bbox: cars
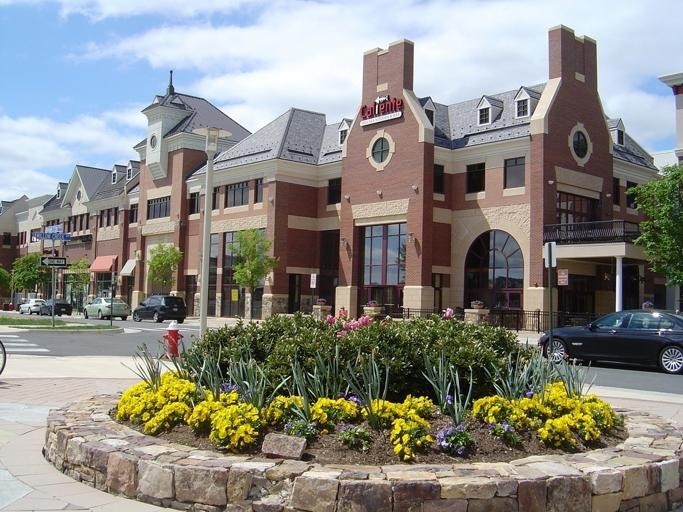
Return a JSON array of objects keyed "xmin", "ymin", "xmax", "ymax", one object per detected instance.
[
  {"xmin": 17, "ymin": 299, "xmax": 45, "ymax": 314},
  {"xmin": 40, "ymin": 298, "xmax": 71, "ymax": 316},
  {"xmin": 536, "ymin": 308, "xmax": 683, "ymax": 373},
  {"xmin": 82, "ymin": 295, "xmax": 127, "ymax": 322}
]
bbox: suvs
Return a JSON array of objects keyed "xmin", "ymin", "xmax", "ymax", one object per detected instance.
[{"xmin": 132, "ymin": 295, "xmax": 186, "ymax": 324}]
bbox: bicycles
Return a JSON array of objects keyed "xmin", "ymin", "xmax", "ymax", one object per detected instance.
[{"xmin": 0, "ymin": 339, "xmax": 5, "ymax": 376}]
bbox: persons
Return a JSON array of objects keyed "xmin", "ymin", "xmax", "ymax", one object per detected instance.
[{"xmin": 641, "ymin": 296, "xmax": 654, "ymax": 310}]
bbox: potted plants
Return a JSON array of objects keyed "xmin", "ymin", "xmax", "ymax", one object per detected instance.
[
  {"xmin": 368, "ymin": 301, "xmax": 378, "ymax": 306},
  {"xmin": 471, "ymin": 300, "xmax": 484, "ymax": 309},
  {"xmin": 316, "ymin": 299, "xmax": 327, "ymax": 306}
]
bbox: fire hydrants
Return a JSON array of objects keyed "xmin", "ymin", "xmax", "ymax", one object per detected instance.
[
  {"xmin": 163, "ymin": 319, "xmax": 183, "ymax": 357},
  {"xmin": 7, "ymin": 302, "xmax": 13, "ymax": 313}
]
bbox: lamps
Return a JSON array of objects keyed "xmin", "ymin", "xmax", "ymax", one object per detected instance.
[
  {"xmin": 408, "ymin": 231, "xmax": 416, "ymax": 243},
  {"xmin": 340, "ymin": 237, "xmax": 348, "ymax": 247}
]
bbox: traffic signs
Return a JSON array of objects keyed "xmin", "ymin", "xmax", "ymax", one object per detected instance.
[{"xmin": 40, "ymin": 257, "xmax": 65, "ymax": 267}]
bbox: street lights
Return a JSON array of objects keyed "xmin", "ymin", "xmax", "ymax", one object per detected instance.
[{"xmin": 192, "ymin": 124, "xmax": 234, "ymax": 341}]
